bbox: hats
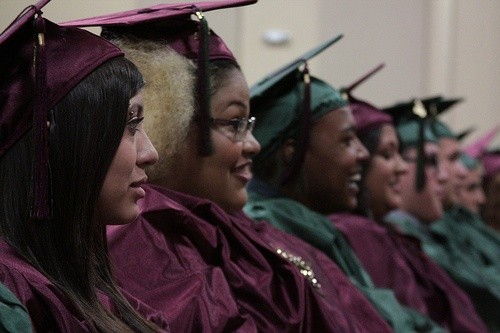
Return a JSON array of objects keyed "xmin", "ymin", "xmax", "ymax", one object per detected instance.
[{"xmin": 1, "ymin": 0, "xmax": 500, "ymax": 185}]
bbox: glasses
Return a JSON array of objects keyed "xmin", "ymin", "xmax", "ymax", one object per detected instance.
[{"xmin": 208, "ymin": 116, "xmax": 257, "ymax": 134}]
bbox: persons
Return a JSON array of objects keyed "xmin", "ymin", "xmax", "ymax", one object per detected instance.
[
  {"xmin": 243, "ymin": 35, "xmax": 500, "ymax": 333},
  {"xmin": 58, "ymin": 0, "xmax": 392, "ymax": 333},
  {"xmin": 0, "ymin": 0, "xmax": 172, "ymax": 333}
]
